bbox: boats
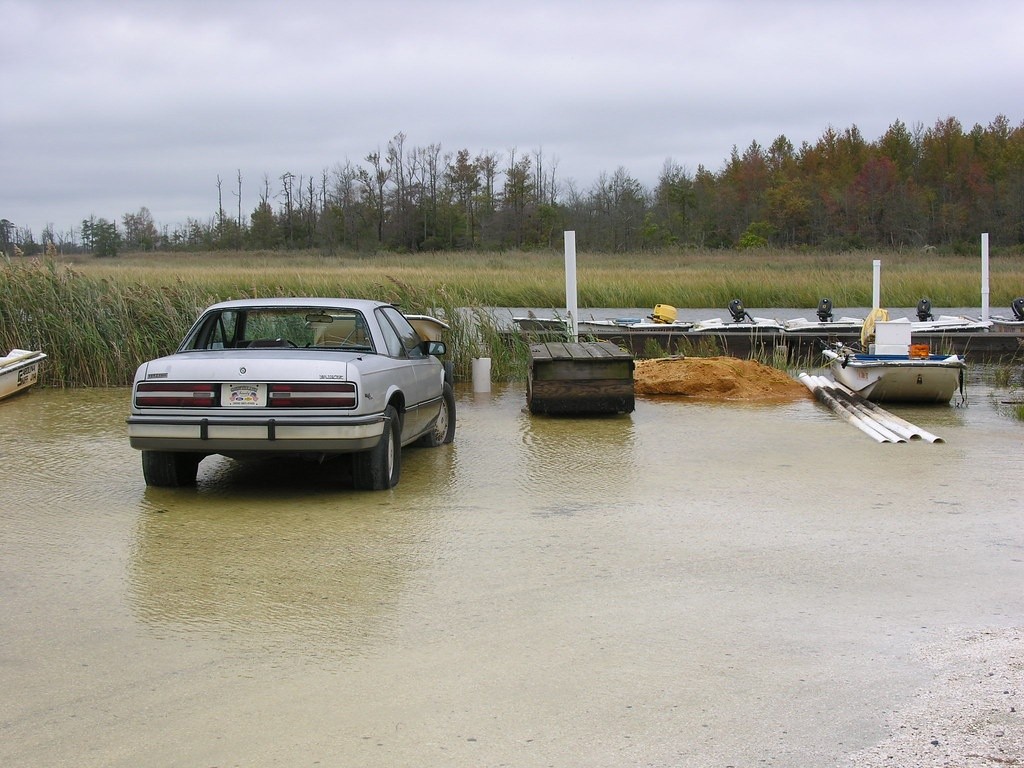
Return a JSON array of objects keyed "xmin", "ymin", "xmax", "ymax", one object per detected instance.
[
  {"xmin": 511, "ymin": 300, "xmax": 696, "ymax": 332},
  {"xmin": 691, "ymin": 297, "xmax": 785, "ymax": 333},
  {"xmin": 977, "ymin": 297, "xmax": 1024, "ymax": 333},
  {"xmin": 306, "ymin": 313, "xmax": 453, "ymax": 347},
  {"xmin": 781, "ymin": 297, "xmax": 866, "ymax": 334},
  {"xmin": 0, "ymin": 347, "xmax": 48, "ymax": 400},
  {"xmin": 910, "ymin": 298, "xmax": 994, "ymax": 333},
  {"xmin": 820, "ymin": 317, "xmax": 968, "ymax": 404}
]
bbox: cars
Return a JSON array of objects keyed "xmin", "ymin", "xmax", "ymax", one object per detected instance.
[{"xmin": 122, "ymin": 295, "xmax": 458, "ymax": 492}]
